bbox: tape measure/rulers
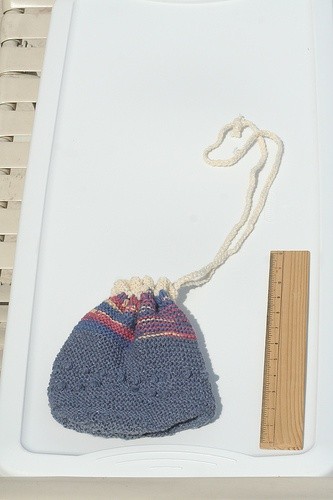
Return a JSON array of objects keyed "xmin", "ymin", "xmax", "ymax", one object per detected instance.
[{"xmin": 259, "ymin": 249, "xmax": 312, "ymax": 451}]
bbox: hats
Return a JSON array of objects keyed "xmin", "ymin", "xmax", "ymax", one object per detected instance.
[{"xmin": 45, "ymin": 112, "xmax": 284, "ymax": 438}]
